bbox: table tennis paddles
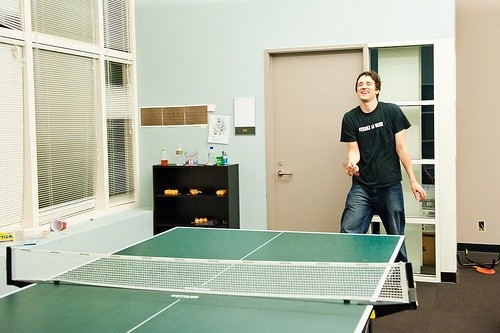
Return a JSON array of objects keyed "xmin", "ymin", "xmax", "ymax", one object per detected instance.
[
  {"xmin": 343, "ymin": 162, "xmax": 360, "ymax": 176},
  {"xmin": 473, "ymin": 266, "xmax": 496, "ymax": 274}
]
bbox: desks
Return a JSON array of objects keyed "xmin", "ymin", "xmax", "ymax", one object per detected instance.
[{"xmin": 0, "ymin": 226, "xmax": 419, "ymax": 333}]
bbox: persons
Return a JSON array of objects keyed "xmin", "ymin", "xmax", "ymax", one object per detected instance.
[{"xmin": 338, "ymin": 71, "xmax": 427, "ymax": 307}]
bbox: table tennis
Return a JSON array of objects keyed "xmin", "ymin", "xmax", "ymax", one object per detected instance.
[
  {"xmin": 165, "ymin": 190, "xmax": 178, "ymax": 195},
  {"xmin": 195, "ymin": 218, "xmax": 208, "ymax": 223},
  {"xmin": 216, "ymin": 189, "xmax": 226, "ymax": 196},
  {"xmin": 190, "ymin": 189, "xmax": 198, "ymax": 195}
]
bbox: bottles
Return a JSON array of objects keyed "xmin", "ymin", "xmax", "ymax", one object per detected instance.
[
  {"xmin": 175, "ymin": 145, "xmax": 184, "ymax": 166},
  {"xmin": 160, "ymin": 147, "xmax": 168, "ymax": 166},
  {"xmin": 208, "ymin": 146, "xmax": 216, "ymax": 165}
]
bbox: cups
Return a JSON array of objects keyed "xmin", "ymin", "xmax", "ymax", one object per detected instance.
[{"xmin": 50, "ymin": 219, "xmax": 67, "ymax": 232}]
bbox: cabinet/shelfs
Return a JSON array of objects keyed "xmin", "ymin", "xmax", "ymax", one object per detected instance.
[{"xmin": 153, "ymin": 164, "xmax": 240, "ymax": 235}]
